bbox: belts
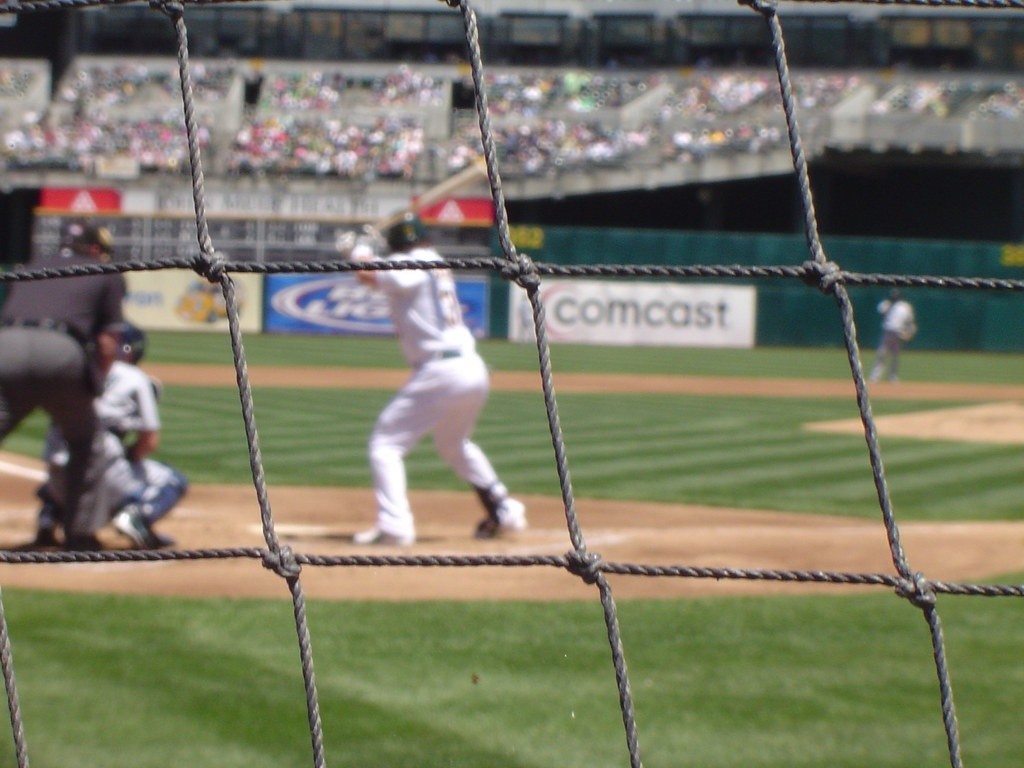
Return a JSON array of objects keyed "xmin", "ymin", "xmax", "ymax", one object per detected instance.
[
  {"xmin": 443, "ymin": 349, "xmax": 460, "ymax": 359},
  {"xmin": 0, "ymin": 318, "xmax": 87, "ymax": 349}
]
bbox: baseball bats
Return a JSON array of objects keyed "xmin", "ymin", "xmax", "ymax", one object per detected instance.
[{"xmin": 374, "ymin": 154, "xmax": 498, "ymax": 232}]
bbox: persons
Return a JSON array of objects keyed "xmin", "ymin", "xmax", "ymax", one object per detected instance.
[
  {"xmin": 870, "ymin": 288, "xmax": 918, "ymax": 384},
  {"xmin": 347, "ymin": 212, "xmax": 525, "ymax": 550},
  {"xmin": 0, "ymin": 216, "xmax": 128, "ymax": 549},
  {"xmin": 0, "ymin": 62, "xmax": 236, "ymax": 174},
  {"xmin": 33, "ymin": 319, "xmax": 190, "ymax": 550},
  {"xmin": 797, "ymin": 74, "xmax": 1023, "ymax": 123},
  {"xmin": 449, "ymin": 70, "xmax": 800, "ymax": 183},
  {"xmin": 232, "ymin": 63, "xmax": 447, "ymax": 184}
]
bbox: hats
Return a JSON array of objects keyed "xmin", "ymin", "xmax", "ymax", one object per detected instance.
[{"xmin": 60, "ymin": 220, "xmax": 113, "ymax": 264}]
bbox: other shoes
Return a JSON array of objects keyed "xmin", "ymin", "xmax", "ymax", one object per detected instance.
[
  {"xmin": 114, "ymin": 502, "xmax": 172, "ymax": 550},
  {"xmin": 35, "ymin": 523, "xmax": 66, "ymax": 545},
  {"xmin": 476, "ymin": 513, "xmax": 526, "ymax": 539},
  {"xmin": 66, "ymin": 522, "xmax": 136, "ymax": 551},
  {"xmin": 354, "ymin": 526, "xmax": 415, "ymax": 546}
]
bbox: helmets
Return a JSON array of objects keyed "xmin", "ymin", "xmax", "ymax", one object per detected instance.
[
  {"xmin": 119, "ymin": 326, "xmax": 144, "ymax": 366},
  {"xmin": 387, "ymin": 216, "xmax": 425, "ymax": 250}
]
